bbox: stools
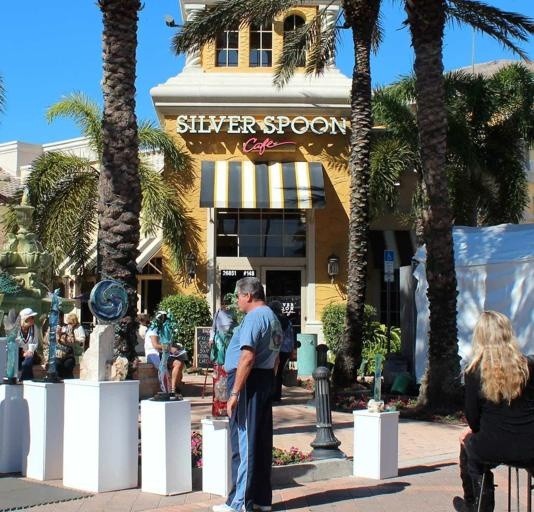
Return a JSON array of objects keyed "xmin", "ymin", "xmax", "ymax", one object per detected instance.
[{"xmin": 476, "ymin": 462, "xmax": 533, "ymax": 511}]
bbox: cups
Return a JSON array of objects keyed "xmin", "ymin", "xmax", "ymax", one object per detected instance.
[{"xmin": 28, "ymin": 344, "xmax": 35, "ymax": 352}]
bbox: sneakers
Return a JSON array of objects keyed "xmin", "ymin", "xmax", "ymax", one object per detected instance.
[{"xmin": 212, "ymin": 503, "xmax": 272, "ymax": 512}]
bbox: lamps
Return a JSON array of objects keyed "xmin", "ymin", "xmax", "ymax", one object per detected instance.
[
  {"xmin": 184, "ymin": 252, "xmax": 197, "ymax": 284},
  {"xmin": 327, "ymin": 252, "xmax": 339, "ymax": 284}
]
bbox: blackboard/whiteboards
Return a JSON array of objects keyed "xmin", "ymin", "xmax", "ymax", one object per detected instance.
[{"xmin": 195, "ymin": 326, "xmax": 215, "ymax": 371}]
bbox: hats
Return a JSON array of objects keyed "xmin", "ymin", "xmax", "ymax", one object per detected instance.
[{"xmin": 19, "ymin": 308, "xmax": 38, "ymax": 319}]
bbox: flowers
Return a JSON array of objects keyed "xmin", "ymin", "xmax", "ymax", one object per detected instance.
[
  {"xmin": 272, "ymin": 444, "xmax": 314, "ymax": 467},
  {"xmin": 191, "ymin": 429, "xmax": 202, "ymax": 469}
]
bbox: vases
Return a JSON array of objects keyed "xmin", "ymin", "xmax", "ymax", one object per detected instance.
[{"xmin": 270, "ymin": 464, "xmax": 315, "ymax": 486}]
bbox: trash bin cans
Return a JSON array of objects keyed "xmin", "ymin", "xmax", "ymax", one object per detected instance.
[{"xmin": 296, "ymin": 333, "xmax": 317, "ymax": 377}]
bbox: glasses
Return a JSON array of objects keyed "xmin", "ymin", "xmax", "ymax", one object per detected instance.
[
  {"xmin": 24, "ymin": 317, "xmax": 33, "ymax": 323},
  {"xmin": 236, "ymin": 294, "xmax": 245, "ymax": 298}
]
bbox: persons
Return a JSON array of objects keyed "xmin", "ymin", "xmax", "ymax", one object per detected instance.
[
  {"xmin": 212, "ymin": 276, "xmax": 283, "ymax": 512},
  {"xmin": 144, "ymin": 310, "xmax": 184, "ymax": 394},
  {"xmin": 453, "ymin": 311, "xmax": 534, "ymax": 512},
  {"xmin": 209, "ymin": 293, "xmax": 294, "ymax": 417},
  {"xmin": 15, "ymin": 308, "xmax": 86, "ymax": 380}
]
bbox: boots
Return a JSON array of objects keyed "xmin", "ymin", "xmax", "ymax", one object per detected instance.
[{"xmin": 453, "ymin": 471, "xmax": 494, "ymax": 512}]
